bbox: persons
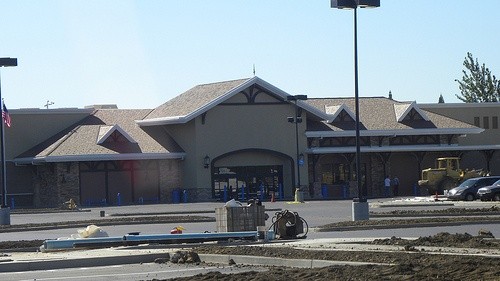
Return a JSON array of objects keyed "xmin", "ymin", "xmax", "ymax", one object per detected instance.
[
  {"xmin": 393, "ymin": 174, "xmax": 400, "ymax": 196},
  {"xmin": 384, "ymin": 175, "xmax": 394, "ymax": 197}
]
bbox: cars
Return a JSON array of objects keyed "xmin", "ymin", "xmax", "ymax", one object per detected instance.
[{"xmin": 476, "ymin": 180, "xmax": 500, "ymax": 201}]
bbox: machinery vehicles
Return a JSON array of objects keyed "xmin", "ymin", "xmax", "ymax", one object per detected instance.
[{"xmin": 418, "ymin": 156, "xmax": 492, "ymax": 194}]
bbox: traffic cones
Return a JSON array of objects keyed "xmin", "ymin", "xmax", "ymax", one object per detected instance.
[{"xmin": 271, "ymin": 194, "xmax": 275, "ymax": 202}]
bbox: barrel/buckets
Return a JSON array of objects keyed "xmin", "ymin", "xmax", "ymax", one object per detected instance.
[{"xmin": 256, "ymin": 225, "xmax": 268, "ymax": 243}]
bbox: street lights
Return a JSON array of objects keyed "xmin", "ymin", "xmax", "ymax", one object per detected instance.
[
  {"xmin": 330, "ymin": 0, "xmax": 383, "ymax": 203},
  {"xmin": 287, "ymin": 95, "xmax": 308, "ymax": 191},
  {"xmin": 0, "ymin": 57, "xmax": 18, "ymax": 207}
]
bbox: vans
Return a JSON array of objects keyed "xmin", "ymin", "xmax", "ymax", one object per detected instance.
[{"xmin": 446, "ymin": 176, "xmax": 500, "ymax": 201}]
